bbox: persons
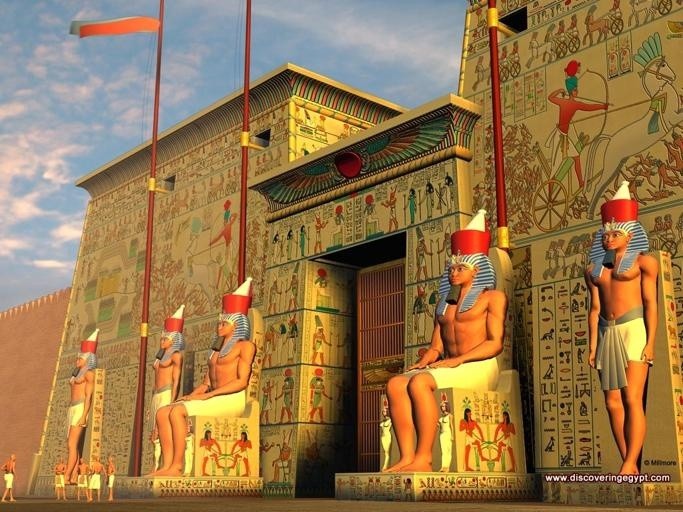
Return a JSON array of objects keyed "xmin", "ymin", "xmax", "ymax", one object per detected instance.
[
  {"xmin": 489, "ymin": 399, "xmax": 518, "ymax": 474},
  {"xmin": 403, "ymin": 478, "xmax": 412, "ymax": 500},
  {"xmin": 581, "ymin": 178, "xmax": 660, "ymax": 484},
  {"xmin": 435, "ymin": 398, "xmax": 454, "ymax": 472},
  {"xmin": 149, "ymin": 304, "xmax": 186, "ymax": 472},
  {"xmin": 377, "ymin": 407, "xmax": 393, "ymax": 474},
  {"xmin": 362, "ymin": 194, "xmax": 383, "ymax": 234},
  {"xmin": 106, "ymin": 454, "xmax": 116, "ymax": 501},
  {"xmin": 54, "ymin": 456, "xmax": 68, "ymax": 501},
  {"xmin": 58, "ymin": 327, "xmax": 100, "ymax": 488},
  {"xmin": 435, "ymin": 224, "xmax": 452, "ymax": 273},
  {"xmin": 540, "ymin": 58, "xmax": 609, "ymax": 192},
  {"xmin": 256, "ymin": 203, "xmax": 356, "ymax": 488},
  {"xmin": 76, "ymin": 457, "xmax": 89, "ymax": 502},
  {"xmin": 0, "ymin": 454, "xmax": 17, "ymax": 503},
  {"xmin": 198, "ymin": 430, "xmax": 223, "ymax": 479},
  {"xmin": 419, "ymin": 183, "xmax": 434, "ymax": 220},
  {"xmin": 229, "ymin": 431, "xmax": 252, "ymax": 478},
  {"xmin": 140, "ymin": 274, "xmax": 258, "ymax": 476},
  {"xmin": 412, "ymin": 283, "xmax": 434, "ymax": 345},
  {"xmin": 458, "ymin": 396, "xmax": 489, "ymax": 472},
  {"xmin": 402, "ymin": 188, "xmax": 418, "ymax": 226},
  {"xmin": 378, "ymin": 206, "xmax": 510, "ymax": 477},
  {"xmin": 442, "ymin": 176, "xmax": 453, "ymax": 213},
  {"xmin": 413, "ymin": 226, "xmax": 433, "ymax": 284},
  {"xmin": 86, "ymin": 456, "xmax": 106, "ymax": 502},
  {"xmin": 208, "ymin": 198, "xmax": 239, "ymax": 292}
]
